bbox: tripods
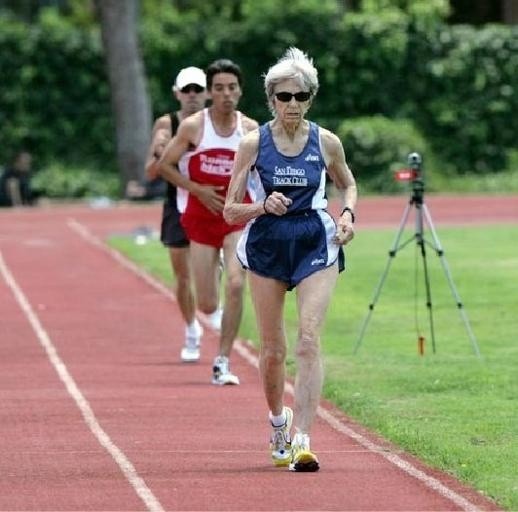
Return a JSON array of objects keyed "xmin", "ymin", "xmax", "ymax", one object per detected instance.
[{"xmin": 352, "ymin": 181, "xmax": 484, "ymax": 365}]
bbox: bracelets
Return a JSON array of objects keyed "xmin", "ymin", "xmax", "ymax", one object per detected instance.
[
  {"xmin": 263, "ymin": 197, "xmax": 269, "ymax": 214},
  {"xmin": 337, "ymin": 206, "xmax": 356, "ymax": 225}
]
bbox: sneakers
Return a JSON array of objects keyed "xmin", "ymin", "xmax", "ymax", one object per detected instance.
[
  {"xmin": 288, "ymin": 446, "xmax": 319, "ymax": 472},
  {"xmin": 181, "ymin": 322, "xmax": 201, "ymax": 361},
  {"xmin": 269, "ymin": 407, "xmax": 294, "ymax": 466},
  {"xmin": 212, "ymin": 363, "xmax": 239, "ymax": 385}
]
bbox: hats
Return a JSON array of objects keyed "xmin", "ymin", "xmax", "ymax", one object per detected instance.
[{"xmin": 176, "ymin": 67, "xmax": 207, "ymax": 90}]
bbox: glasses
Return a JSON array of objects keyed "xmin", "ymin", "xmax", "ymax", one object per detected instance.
[
  {"xmin": 181, "ymin": 86, "xmax": 203, "ymax": 92},
  {"xmin": 274, "ymin": 92, "xmax": 311, "ymax": 101}
]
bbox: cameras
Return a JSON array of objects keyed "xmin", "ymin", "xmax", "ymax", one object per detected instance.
[{"xmin": 397, "ymin": 168, "xmax": 423, "ymax": 181}]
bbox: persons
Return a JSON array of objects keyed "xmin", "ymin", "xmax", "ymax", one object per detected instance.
[
  {"xmin": 223, "ymin": 46, "xmax": 359, "ymax": 473},
  {"xmin": 144, "ymin": 65, "xmax": 208, "ymax": 364},
  {"xmin": 156, "ymin": 58, "xmax": 266, "ymax": 387}
]
